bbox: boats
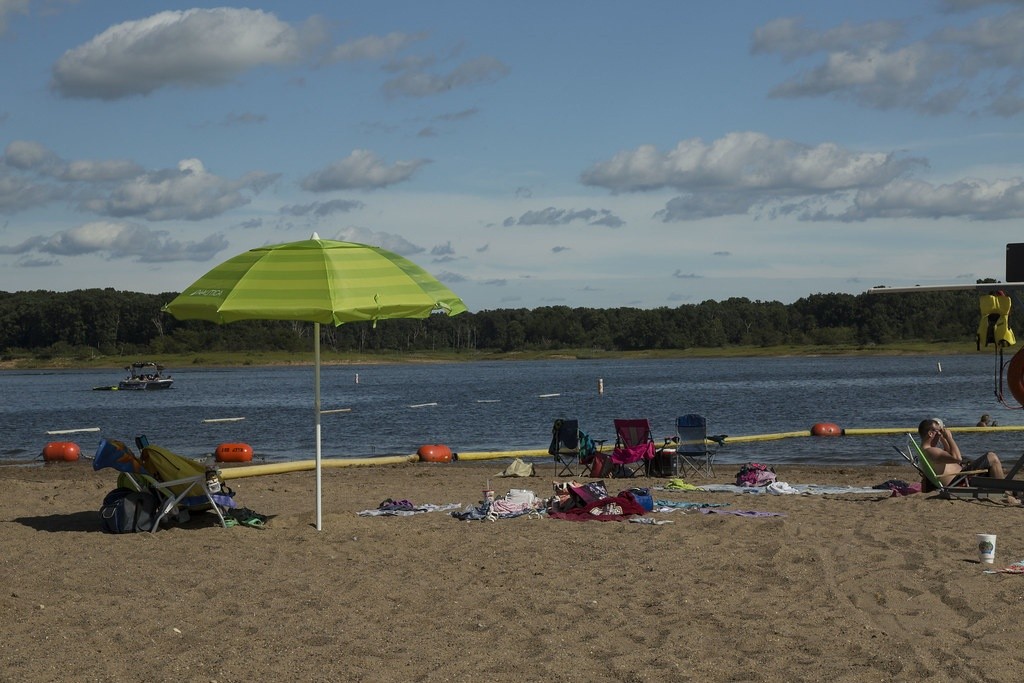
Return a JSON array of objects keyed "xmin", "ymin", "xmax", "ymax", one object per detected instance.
[{"xmin": 119, "ymin": 361, "xmax": 173, "ymax": 389}]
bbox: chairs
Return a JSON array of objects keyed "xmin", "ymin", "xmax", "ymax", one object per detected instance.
[
  {"xmin": 93, "ymin": 437, "xmax": 236, "ymax": 535},
  {"xmin": 547, "ymin": 414, "xmax": 728, "ymax": 480},
  {"xmin": 892, "ymin": 431, "xmax": 989, "ymax": 494}
]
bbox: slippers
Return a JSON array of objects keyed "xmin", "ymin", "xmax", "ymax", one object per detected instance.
[
  {"xmin": 398, "ymin": 500, "xmax": 421, "ymax": 512},
  {"xmin": 378, "ymin": 499, "xmax": 400, "ymax": 511}
]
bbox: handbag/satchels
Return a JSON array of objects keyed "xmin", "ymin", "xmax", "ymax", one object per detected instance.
[
  {"xmin": 100, "ymin": 486, "xmax": 155, "ymax": 533},
  {"xmin": 736, "ymin": 463, "xmax": 777, "ymax": 489},
  {"xmin": 496, "ymin": 458, "xmax": 535, "ymax": 477},
  {"xmin": 619, "ymin": 487, "xmax": 654, "ymax": 512}
]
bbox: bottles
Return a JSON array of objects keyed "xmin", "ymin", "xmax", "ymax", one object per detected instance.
[
  {"xmin": 154, "ymin": 494, "xmax": 175, "ymax": 521},
  {"xmin": 205, "ymin": 471, "xmax": 220, "ymax": 494},
  {"xmin": 204, "ymin": 453, "xmax": 223, "ymax": 485}
]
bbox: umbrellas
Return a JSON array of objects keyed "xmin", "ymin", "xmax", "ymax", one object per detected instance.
[{"xmin": 159, "ymin": 232, "xmax": 467, "ymax": 531}]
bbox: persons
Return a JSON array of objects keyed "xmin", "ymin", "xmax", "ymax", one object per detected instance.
[
  {"xmin": 977, "ymin": 414, "xmax": 989, "ymax": 427},
  {"xmin": 918, "ymin": 420, "xmax": 1021, "ymax": 505}
]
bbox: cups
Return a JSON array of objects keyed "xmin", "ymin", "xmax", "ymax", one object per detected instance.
[
  {"xmin": 482, "ymin": 489, "xmax": 495, "ymax": 507},
  {"xmin": 976, "ymin": 534, "xmax": 997, "ymax": 563}
]
